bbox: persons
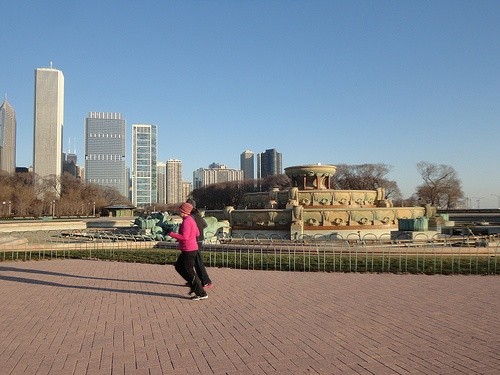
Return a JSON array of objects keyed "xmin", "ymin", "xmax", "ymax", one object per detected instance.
[
  {"xmin": 169, "ymin": 203, "xmax": 208, "ymax": 300},
  {"xmin": 186, "ymin": 198, "xmax": 213, "ymax": 288}
]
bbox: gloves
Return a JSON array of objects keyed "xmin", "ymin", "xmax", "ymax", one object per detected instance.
[{"xmin": 169, "ymin": 231, "xmax": 174, "ymax": 237}]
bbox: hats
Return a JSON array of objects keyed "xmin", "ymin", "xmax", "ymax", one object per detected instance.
[
  {"xmin": 186, "ymin": 199, "xmax": 197, "ymax": 209},
  {"xmin": 178, "ymin": 203, "xmax": 193, "ymax": 215}
]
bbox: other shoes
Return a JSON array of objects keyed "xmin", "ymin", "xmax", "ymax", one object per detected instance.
[
  {"xmin": 190, "ymin": 293, "xmax": 208, "ymax": 301},
  {"xmin": 202, "ymin": 282, "xmax": 212, "ymax": 288},
  {"xmin": 189, "ymin": 289, "xmax": 195, "ymax": 295}
]
